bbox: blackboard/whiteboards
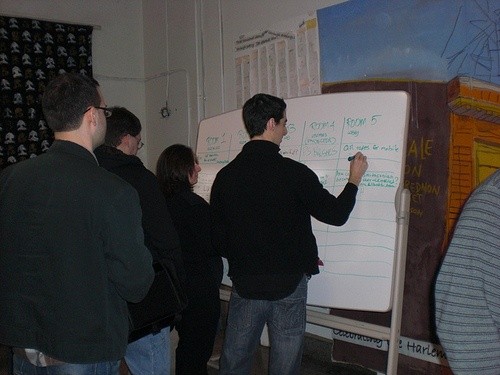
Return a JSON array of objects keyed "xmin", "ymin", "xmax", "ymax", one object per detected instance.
[{"xmin": 193, "ymin": 90, "xmax": 410, "ymax": 313}]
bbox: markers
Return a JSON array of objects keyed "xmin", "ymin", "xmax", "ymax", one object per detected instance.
[{"xmin": 348, "ymin": 155, "xmax": 356, "ymax": 161}]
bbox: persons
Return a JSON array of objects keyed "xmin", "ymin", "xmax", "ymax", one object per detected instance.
[
  {"xmin": 0, "ymin": 73, "xmax": 368, "ymax": 375},
  {"xmin": 434, "ymin": 171, "xmax": 500, "ymax": 375}
]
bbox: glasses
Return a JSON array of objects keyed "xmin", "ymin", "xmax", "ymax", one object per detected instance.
[
  {"xmin": 124, "ymin": 133, "xmax": 144, "ymax": 149},
  {"xmin": 83, "ymin": 107, "xmax": 112, "ymax": 118}
]
